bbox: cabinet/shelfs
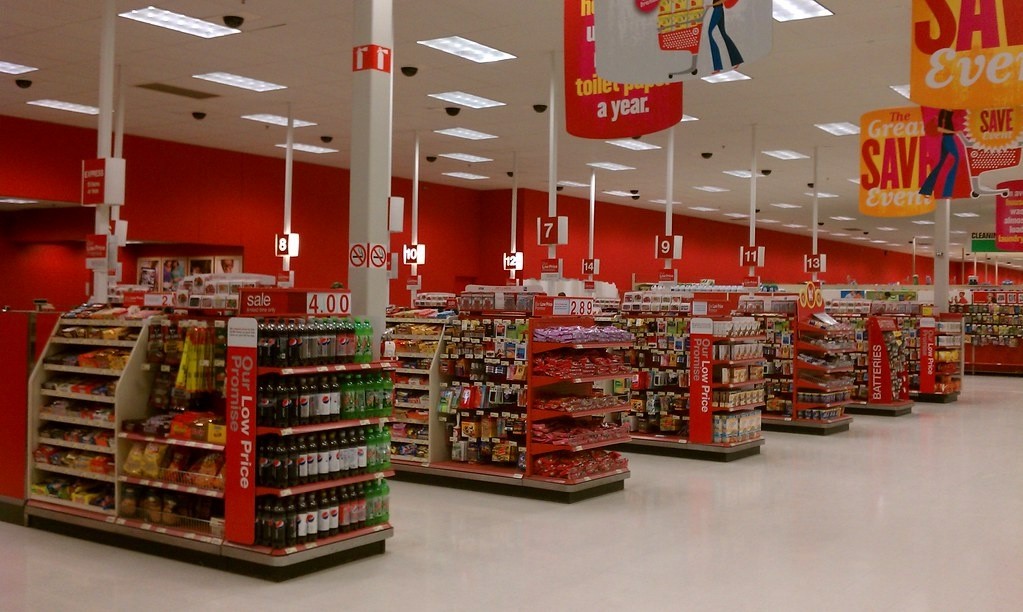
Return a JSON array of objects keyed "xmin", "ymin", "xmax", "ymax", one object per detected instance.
[
  {"xmin": 23, "ymin": 314, "xmax": 395, "ymax": 581},
  {"xmin": 891, "ymin": 313, "xmax": 965, "ymax": 404},
  {"xmin": 738, "ymin": 300, "xmax": 857, "ymax": 437},
  {"xmin": 829, "ymin": 313, "xmax": 915, "ymax": 417},
  {"xmin": 386, "ymin": 316, "xmax": 632, "ymax": 503},
  {"xmin": 594, "ymin": 314, "xmax": 767, "ymax": 463}
]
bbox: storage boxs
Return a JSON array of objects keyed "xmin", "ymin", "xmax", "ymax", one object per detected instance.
[{"xmin": 31, "ymin": 274, "xmax": 764, "ymax": 538}]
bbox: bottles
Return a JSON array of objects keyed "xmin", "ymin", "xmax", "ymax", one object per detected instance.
[{"xmin": 254, "ymin": 317, "xmax": 391, "ymax": 547}]
